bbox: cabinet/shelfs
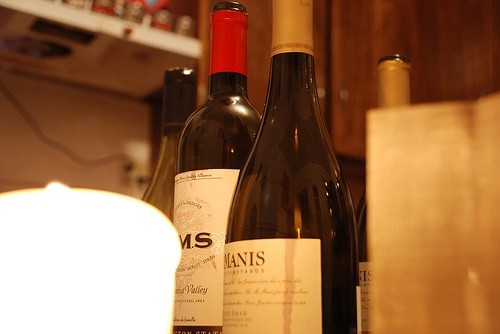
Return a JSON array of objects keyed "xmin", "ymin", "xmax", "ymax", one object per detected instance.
[{"xmin": 212, "ymin": 1, "xmax": 500, "ymax": 169}]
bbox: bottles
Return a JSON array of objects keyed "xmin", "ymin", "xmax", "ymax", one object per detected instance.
[
  {"xmin": 170, "ymin": 2, "xmax": 263, "ymax": 334},
  {"xmin": 140, "ymin": 65, "xmax": 198, "ymax": 223},
  {"xmin": 354, "ymin": 55, "xmax": 413, "ymax": 333},
  {"xmin": 223, "ymin": 1, "xmax": 363, "ymax": 333}
]
